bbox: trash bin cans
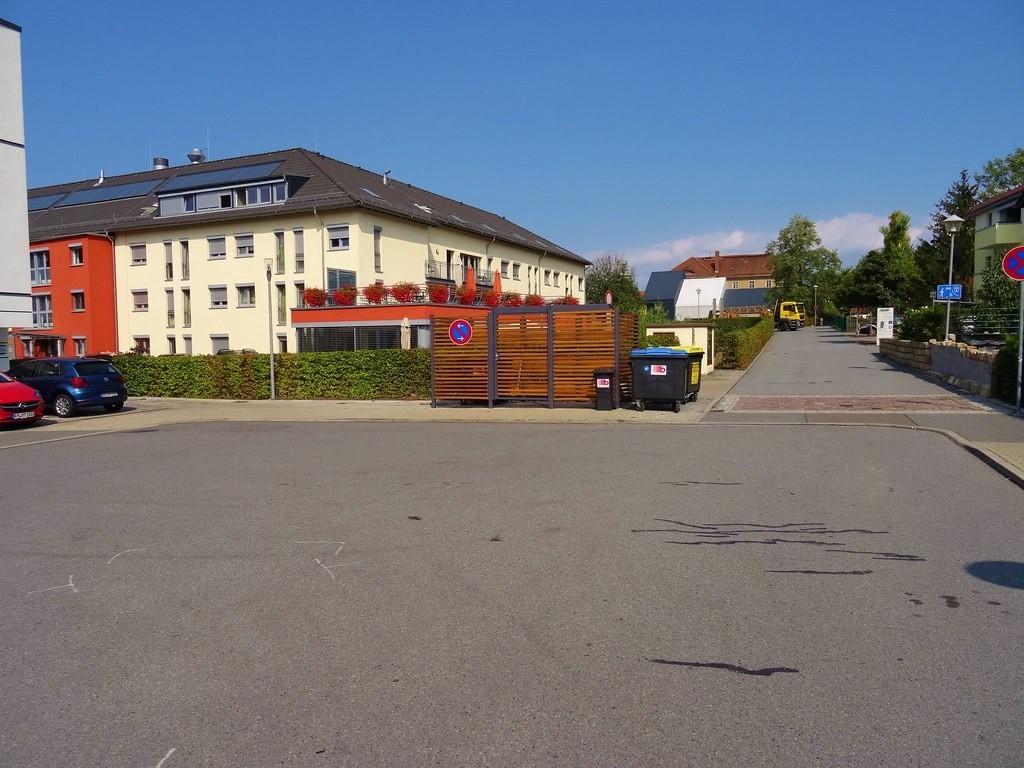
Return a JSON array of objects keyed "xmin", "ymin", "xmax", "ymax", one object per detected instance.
[
  {"xmin": 593, "ymin": 367, "xmax": 616, "ymax": 410},
  {"xmin": 627, "ymin": 349, "xmax": 690, "ymax": 413},
  {"xmin": 660, "ymin": 346, "xmax": 705, "ymax": 402}
]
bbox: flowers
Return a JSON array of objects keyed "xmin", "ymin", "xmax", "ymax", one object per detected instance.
[
  {"xmin": 481, "ymin": 290, "xmax": 501, "ymax": 307},
  {"xmin": 425, "ymin": 283, "xmax": 450, "ymax": 304},
  {"xmin": 363, "ymin": 283, "xmax": 389, "ymax": 304},
  {"xmin": 554, "ymin": 295, "xmax": 580, "ymax": 306},
  {"xmin": 502, "ymin": 291, "xmax": 524, "ymax": 306},
  {"xmin": 333, "ymin": 283, "xmax": 359, "ymax": 307},
  {"xmin": 302, "ymin": 287, "xmax": 329, "ymax": 308},
  {"xmin": 389, "ymin": 282, "xmax": 420, "ymax": 303},
  {"xmin": 524, "ymin": 293, "xmax": 545, "ymax": 306},
  {"xmin": 456, "ymin": 286, "xmax": 477, "ymax": 305}
]
